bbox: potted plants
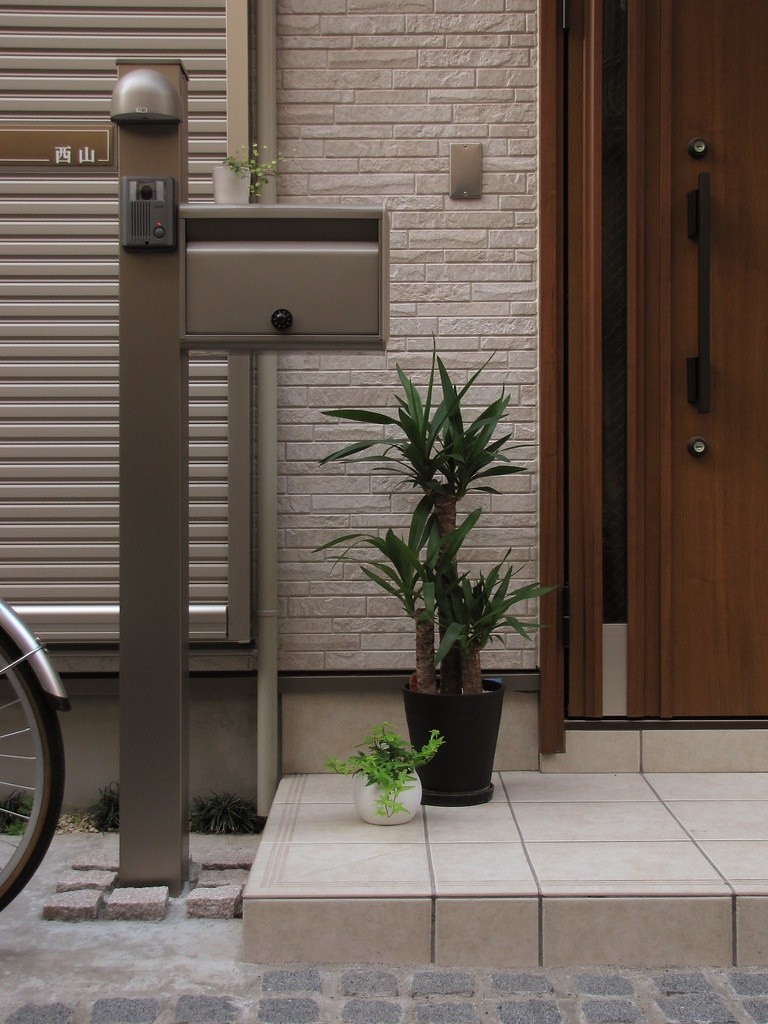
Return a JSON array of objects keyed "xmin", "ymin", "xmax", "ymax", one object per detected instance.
[
  {"xmin": 323, "ymin": 720, "xmax": 448, "ymax": 834},
  {"xmin": 314, "ymin": 350, "xmax": 542, "ymax": 807}
]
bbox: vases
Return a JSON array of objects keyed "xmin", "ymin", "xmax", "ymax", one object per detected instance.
[{"xmin": 214, "ymin": 164, "xmax": 251, "ymax": 207}]
